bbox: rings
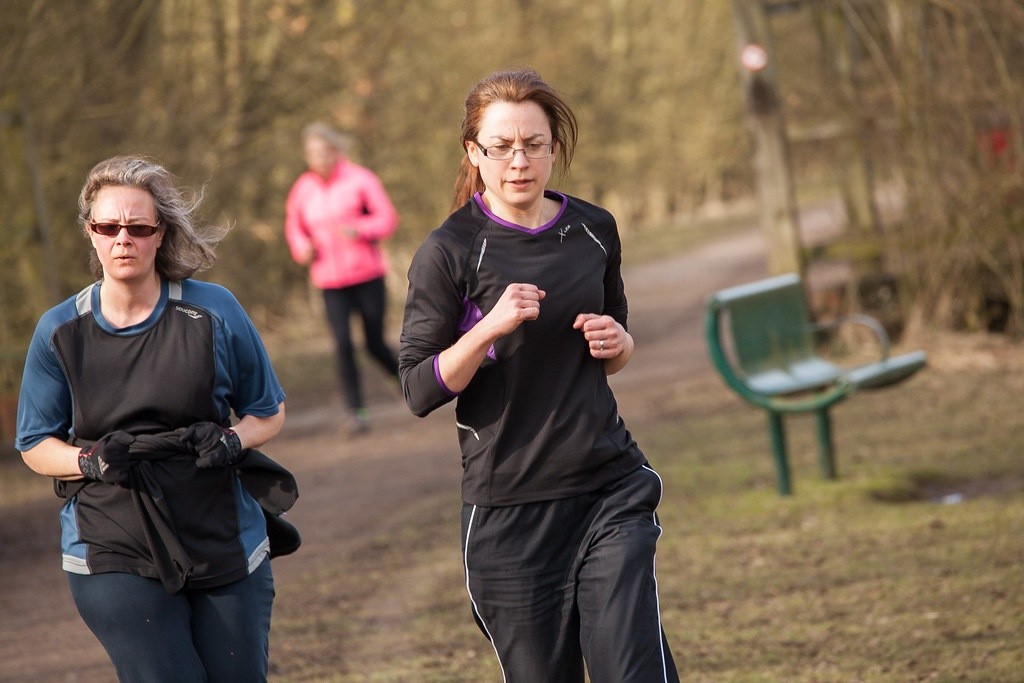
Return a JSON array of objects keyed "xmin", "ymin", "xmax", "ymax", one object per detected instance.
[{"xmin": 600, "ymin": 339, "xmax": 603, "ymax": 349}]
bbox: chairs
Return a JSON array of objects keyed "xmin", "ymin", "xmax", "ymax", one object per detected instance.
[{"xmin": 703, "ymin": 272, "xmax": 929, "ymax": 492}]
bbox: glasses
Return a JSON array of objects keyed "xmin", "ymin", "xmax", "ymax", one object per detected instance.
[
  {"xmin": 472, "ymin": 136, "xmax": 555, "ymax": 160},
  {"xmin": 90, "ymin": 218, "xmax": 161, "ymax": 238}
]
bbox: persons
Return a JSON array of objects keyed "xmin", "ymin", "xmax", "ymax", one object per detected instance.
[
  {"xmin": 284, "ymin": 122, "xmax": 401, "ymax": 431},
  {"xmin": 16, "ymin": 156, "xmax": 304, "ymax": 683},
  {"xmin": 399, "ymin": 79, "xmax": 678, "ymax": 683}
]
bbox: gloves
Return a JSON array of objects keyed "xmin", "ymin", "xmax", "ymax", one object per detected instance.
[
  {"xmin": 77, "ymin": 431, "xmax": 143, "ymax": 483},
  {"xmin": 180, "ymin": 422, "xmax": 241, "ymax": 468}
]
